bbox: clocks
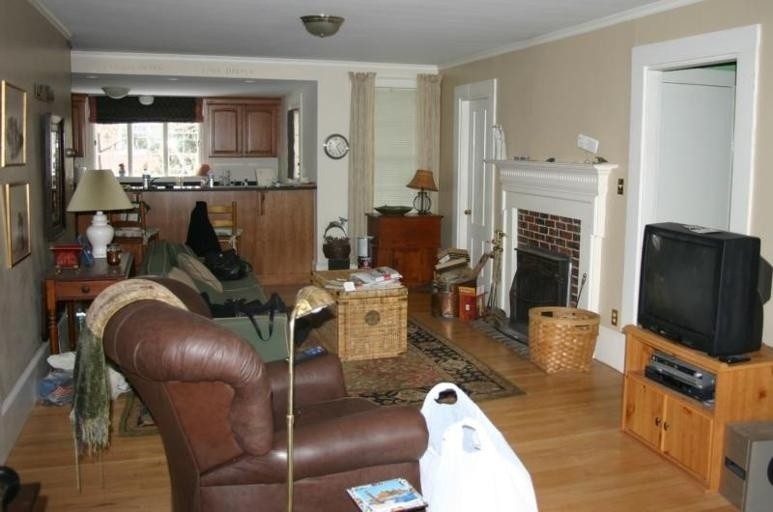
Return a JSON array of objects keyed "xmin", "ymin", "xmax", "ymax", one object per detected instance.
[{"xmin": 325, "ymin": 134, "xmax": 349, "ymax": 159}]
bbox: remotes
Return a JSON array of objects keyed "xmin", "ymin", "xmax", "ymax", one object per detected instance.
[{"xmin": 718, "ymin": 354, "xmax": 751, "ymax": 364}]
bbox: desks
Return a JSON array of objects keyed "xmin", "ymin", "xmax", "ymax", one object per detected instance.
[{"xmin": 43, "ymin": 252, "xmax": 133, "ymax": 371}]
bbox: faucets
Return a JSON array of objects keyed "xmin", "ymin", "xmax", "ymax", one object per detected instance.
[{"xmin": 149, "ymin": 177, "xmax": 160, "ymax": 190}]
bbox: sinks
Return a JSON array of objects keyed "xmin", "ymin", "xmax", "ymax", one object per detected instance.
[
  {"xmin": 170, "ymin": 184, "xmax": 201, "ymax": 191},
  {"xmin": 151, "ymin": 184, "xmax": 170, "ymax": 191}
]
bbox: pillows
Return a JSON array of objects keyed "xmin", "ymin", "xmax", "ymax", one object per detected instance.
[
  {"xmin": 169, "ymin": 240, "xmax": 224, "ymax": 293},
  {"xmin": 167, "ymin": 266, "xmax": 200, "ymax": 294}
]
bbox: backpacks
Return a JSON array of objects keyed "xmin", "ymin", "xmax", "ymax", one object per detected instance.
[{"xmin": 202, "ymin": 248, "xmax": 252, "ymax": 281}]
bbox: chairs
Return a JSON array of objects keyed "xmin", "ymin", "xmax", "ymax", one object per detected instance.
[
  {"xmin": 107, "ymin": 203, "xmax": 159, "ymax": 256},
  {"xmin": 102, "ymin": 276, "xmax": 430, "ymax": 511},
  {"xmin": 207, "ymin": 201, "xmax": 243, "ymax": 256}
]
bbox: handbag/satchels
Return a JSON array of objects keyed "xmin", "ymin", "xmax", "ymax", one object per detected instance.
[{"xmin": 200, "ymin": 291, "xmax": 285, "ymax": 342}]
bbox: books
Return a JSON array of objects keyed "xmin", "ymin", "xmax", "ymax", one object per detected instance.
[{"xmin": 324, "ymin": 264, "xmax": 404, "ymax": 291}]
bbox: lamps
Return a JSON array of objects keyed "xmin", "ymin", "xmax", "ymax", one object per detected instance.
[
  {"xmin": 407, "ymin": 169, "xmax": 438, "ymax": 214},
  {"xmin": 101, "ymin": 87, "xmax": 131, "ymax": 99},
  {"xmin": 300, "ymin": 16, "xmax": 344, "ymax": 38},
  {"xmin": 66, "ymin": 169, "xmax": 133, "ymax": 260},
  {"xmin": 139, "ymin": 97, "xmax": 155, "ymax": 105}
]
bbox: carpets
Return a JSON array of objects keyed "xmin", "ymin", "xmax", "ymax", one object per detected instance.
[{"xmin": 117, "ymin": 306, "xmax": 526, "ymax": 437}]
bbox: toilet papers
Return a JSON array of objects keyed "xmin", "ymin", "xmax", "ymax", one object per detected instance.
[{"xmin": 358, "ymin": 239, "xmax": 369, "ymax": 257}]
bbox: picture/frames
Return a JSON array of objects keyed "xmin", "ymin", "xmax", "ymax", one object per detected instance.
[
  {"xmin": 42, "ymin": 113, "xmax": 67, "ymax": 244},
  {"xmin": 5, "ymin": 181, "xmax": 31, "ymax": 268},
  {"xmin": 2, "ymin": 79, "xmax": 28, "ymax": 167}
]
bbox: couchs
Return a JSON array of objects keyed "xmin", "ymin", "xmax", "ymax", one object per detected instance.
[{"xmin": 139, "ymin": 238, "xmax": 290, "ymax": 362}]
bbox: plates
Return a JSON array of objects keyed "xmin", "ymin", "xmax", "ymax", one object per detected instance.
[{"xmin": 374, "ymin": 205, "xmax": 412, "ymax": 215}]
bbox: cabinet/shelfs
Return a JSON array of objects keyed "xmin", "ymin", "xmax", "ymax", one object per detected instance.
[
  {"xmin": 72, "ymin": 95, "xmax": 91, "ymax": 158},
  {"xmin": 621, "ymin": 325, "xmax": 773, "ymax": 494},
  {"xmin": 364, "ymin": 212, "xmax": 443, "ymax": 289},
  {"xmin": 311, "ymin": 269, "xmax": 408, "ymax": 361},
  {"xmin": 203, "ymin": 98, "xmax": 280, "ymax": 158}
]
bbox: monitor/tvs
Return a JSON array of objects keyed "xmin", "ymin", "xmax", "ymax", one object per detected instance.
[{"xmin": 636, "ymin": 221, "xmax": 772, "ymax": 357}]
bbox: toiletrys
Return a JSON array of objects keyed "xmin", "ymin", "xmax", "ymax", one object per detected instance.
[{"xmin": 142, "ymin": 162, "xmax": 151, "ymax": 190}]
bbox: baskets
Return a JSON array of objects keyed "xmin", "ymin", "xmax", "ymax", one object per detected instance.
[{"xmin": 527, "ymin": 306, "xmax": 599, "ymax": 376}]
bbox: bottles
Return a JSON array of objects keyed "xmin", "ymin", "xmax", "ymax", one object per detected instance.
[{"xmin": 106, "ymin": 243, "xmax": 123, "ymax": 266}]
